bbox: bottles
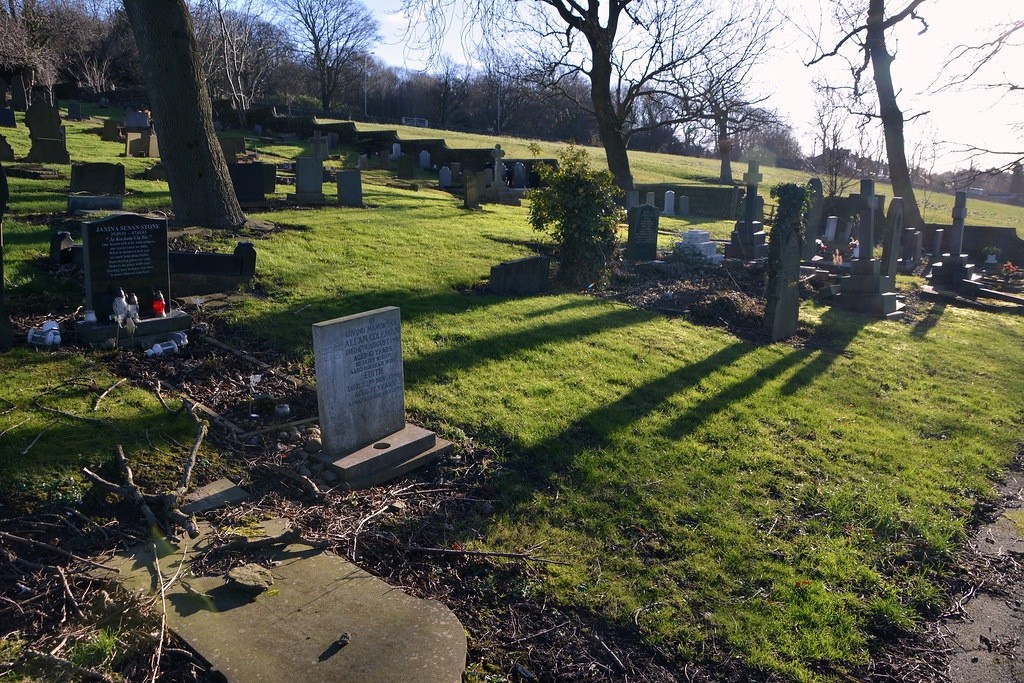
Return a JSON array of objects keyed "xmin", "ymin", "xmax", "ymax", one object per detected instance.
[
  {"xmin": 84, "ymin": 310, "xmax": 97, "ymax": 324},
  {"xmin": 153, "ymin": 291, "xmax": 166, "ymax": 317},
  {"xmin": 127, "ymin": 293, "xmax": 139, "ymax": 314}
]
